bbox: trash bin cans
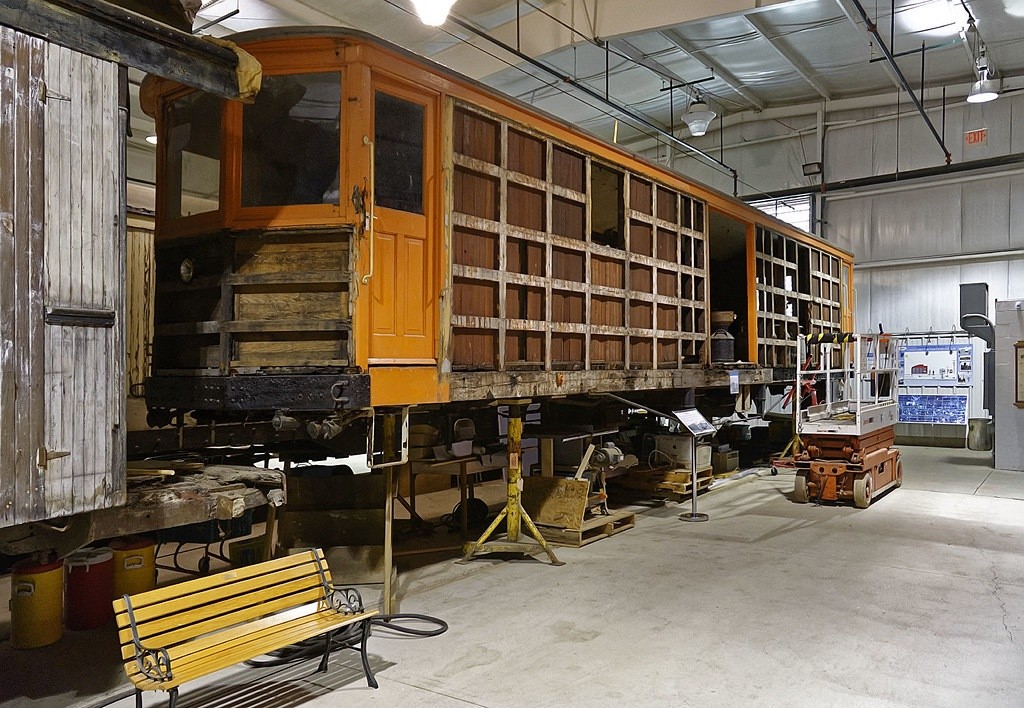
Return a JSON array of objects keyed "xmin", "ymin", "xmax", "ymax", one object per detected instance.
[{"xmin": 968, "ymin": 417, "xmax": 993, "ymax": 451}]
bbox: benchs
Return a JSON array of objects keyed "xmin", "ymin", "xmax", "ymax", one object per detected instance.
[{"xmin": 110, "ymin": 546, "xmax": 380, "ymax": 708}]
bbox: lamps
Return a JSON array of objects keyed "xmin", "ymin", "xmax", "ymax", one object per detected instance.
[
  {"xmin": 681, "ymin": 101, "xmax": 717, "ymax": 136},
  {"xmin": 411, "ymin": 0, "xmax": 458, "ymax": 26},
  {"xmin": 967, "ymin": 80, "xmax": 998, "ymax": 103}
]
